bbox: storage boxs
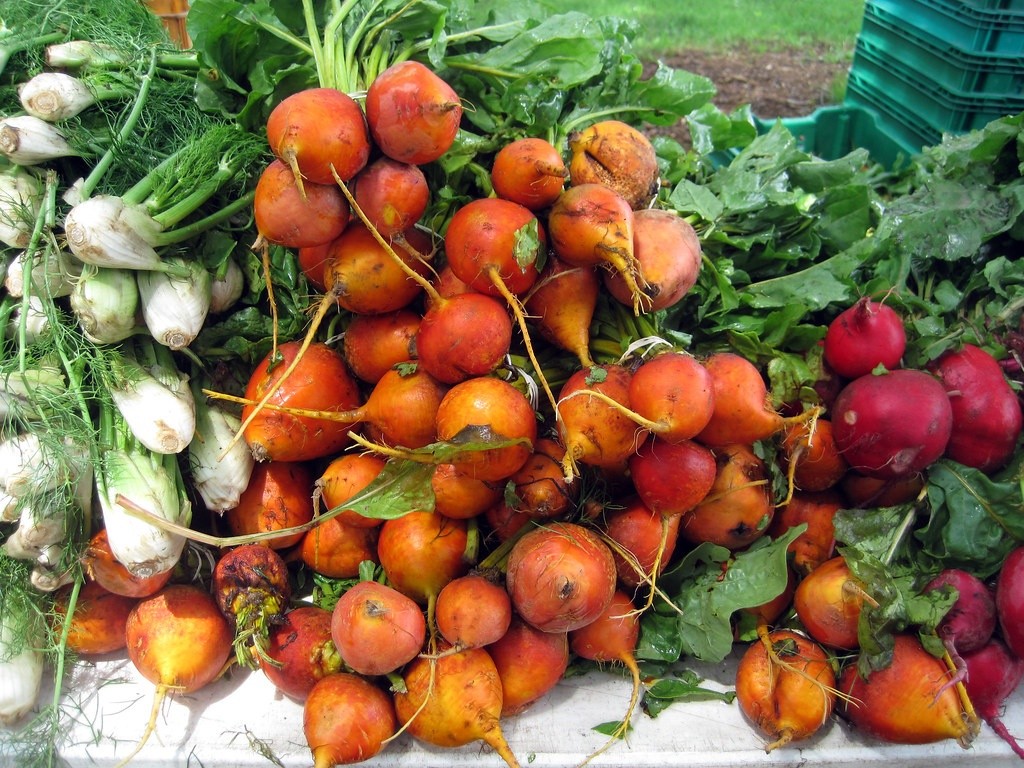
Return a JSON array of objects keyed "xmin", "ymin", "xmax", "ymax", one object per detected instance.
[{"xmin": 706, "ymin": 0, "xmax": 1024, "ymax": 182}]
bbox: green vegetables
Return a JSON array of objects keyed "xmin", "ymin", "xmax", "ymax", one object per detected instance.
[{"xmin": 0, "ymin": 0, "xmax": 301, "ymax": 768}]
